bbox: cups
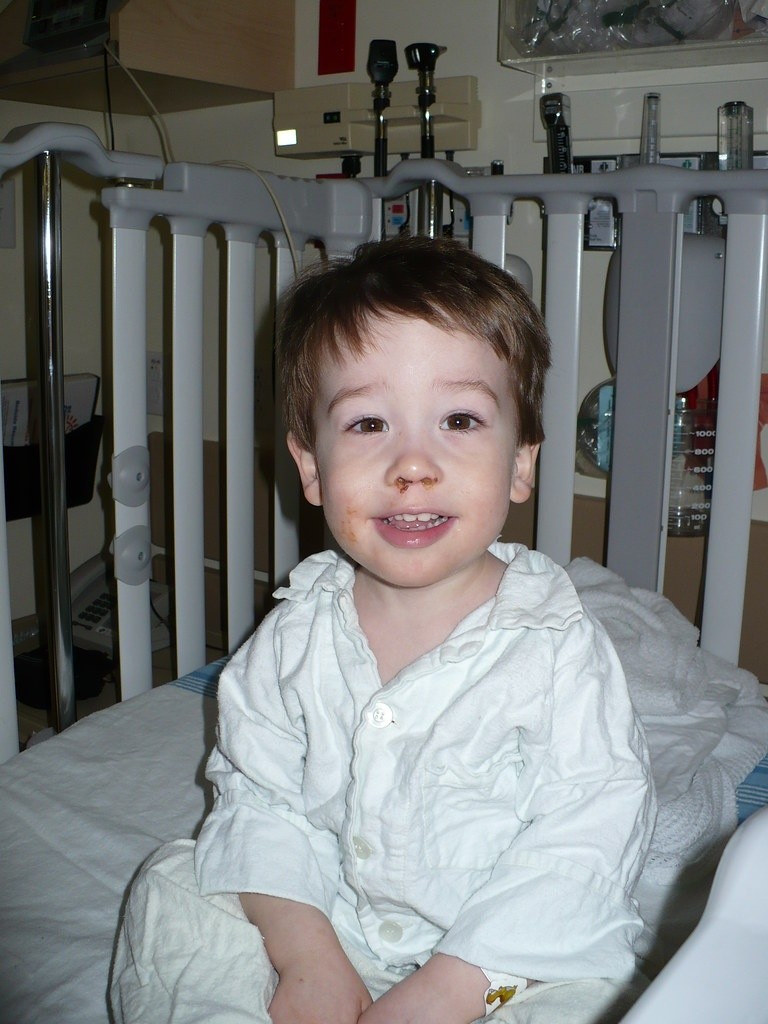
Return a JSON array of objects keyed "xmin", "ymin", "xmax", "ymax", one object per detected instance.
[{"xmin": 668, "ymin": 399, "xmax": 717, "ymax": 537}]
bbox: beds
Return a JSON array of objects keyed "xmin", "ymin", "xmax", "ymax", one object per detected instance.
[{"xmin": 0, "ymin": 123, "xmax": 766, "ymax": 1021}]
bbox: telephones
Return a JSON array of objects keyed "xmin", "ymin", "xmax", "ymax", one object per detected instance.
[{"xmin": 71, "ymin": 548, "xmax": 177, "ymax": 663}]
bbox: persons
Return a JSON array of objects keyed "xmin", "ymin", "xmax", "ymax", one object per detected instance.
[{"xmin": 110, "ymin": 236, "xmax": 656, "ymax": 1024}]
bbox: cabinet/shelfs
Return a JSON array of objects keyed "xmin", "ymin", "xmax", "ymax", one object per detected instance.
[{"xmin": 0, "ymin": 0, "xmax": 294, "ymax": 116}]
